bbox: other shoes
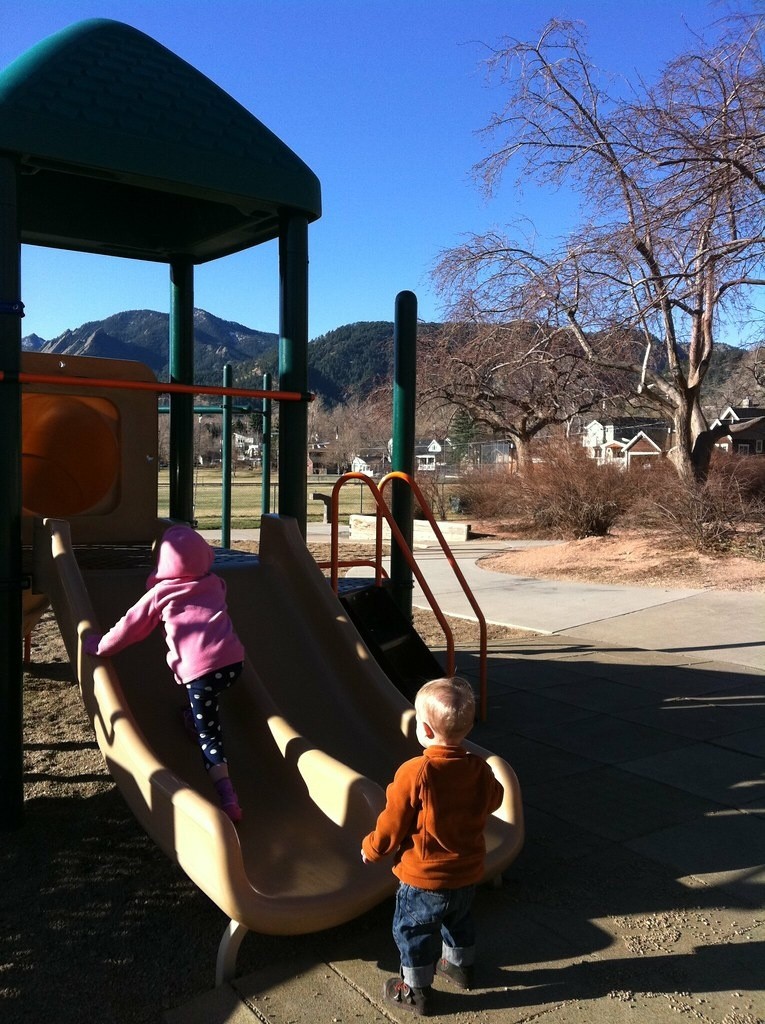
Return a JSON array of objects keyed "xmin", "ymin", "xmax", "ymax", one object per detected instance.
[{"xmin": 222, "ymin": 804, "xmax": 242, "ymax": 823}]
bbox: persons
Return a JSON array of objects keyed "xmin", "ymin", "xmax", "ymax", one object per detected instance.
[
  {"xmin": 361, "ymin": 676, "xmax": 504, "ymax": 1015},
  {"xmin": 80, "ymin": 525, "xmax": 246, "ymax": 823}
]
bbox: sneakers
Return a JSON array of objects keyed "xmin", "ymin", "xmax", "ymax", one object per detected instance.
[
  {"xmin": 383, "ymin": 978, "xmax": 434, "ymax": 1015},
  {"xmin": 435, "ymin": 958, "xmax": 474, "ymax": 988}
]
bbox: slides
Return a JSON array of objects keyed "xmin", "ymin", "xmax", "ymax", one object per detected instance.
[{"xmin": 52, "ymin": 514, "xmax": 524, "ymax": 937}]
